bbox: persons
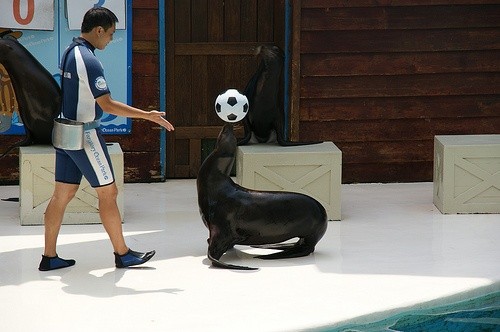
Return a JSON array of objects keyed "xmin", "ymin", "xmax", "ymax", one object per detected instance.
[{"xmin": 37, "ymin": 6, "xmax": 175, "ymax": 272}]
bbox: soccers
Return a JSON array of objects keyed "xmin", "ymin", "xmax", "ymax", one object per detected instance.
[{"xmin": 214, "ymin": 89, "xmax": 249, "ymax": 122}]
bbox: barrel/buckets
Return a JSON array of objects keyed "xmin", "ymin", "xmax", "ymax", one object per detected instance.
[{"xmin": 53, "ymin": 117, "xmax": 85, "ymax": 151}]
